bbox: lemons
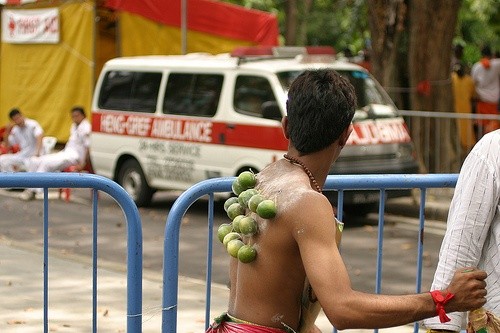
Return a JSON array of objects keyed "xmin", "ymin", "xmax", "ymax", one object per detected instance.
[{"xmin": 217, "ymin": 171, "xmax": 276, "ymax": 263}]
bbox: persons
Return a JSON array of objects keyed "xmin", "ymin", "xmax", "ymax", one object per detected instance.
[
  {"xmin": 0, "ymin": 109, "xmax": 45, "ymax": 176},
  {"xmin": 424, "ymin": 128, "xmax": 500, "ymax": 333},
  {"xmin": 208, "ymin": 68, "xmax": 487, "ymax": 333},
  {"xmin": 449, "ymin": 41, "xmax": 500, "ymax": 154},
  {"xmin": 22, "ymin": 107, "xmax": 92, "ymax": 200}
]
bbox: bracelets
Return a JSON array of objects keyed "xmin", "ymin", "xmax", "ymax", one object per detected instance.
[{"xmin": 430, "ymin": 290, "xmax": 454, "ymax": 323}]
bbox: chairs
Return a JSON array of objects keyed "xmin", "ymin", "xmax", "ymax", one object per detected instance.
[
  {"xmin": 59, "ymin": 148, "xmax": 102, "ymax": 204},
  {"xmin": 10, "ymin": 136, "xmax": 58, "ymax": 171}
]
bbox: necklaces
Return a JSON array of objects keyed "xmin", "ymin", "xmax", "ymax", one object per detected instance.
[{"xmin": 283, "ymin": 153, "xmax": 323, "ymax": 195}]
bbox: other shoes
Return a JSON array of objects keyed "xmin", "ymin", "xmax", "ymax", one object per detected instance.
[{"xmin": 19, "ymin": 190, "xmax": 35, "ymax": 201}]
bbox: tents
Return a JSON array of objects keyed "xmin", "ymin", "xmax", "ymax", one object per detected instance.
[{"xmin": 0, "ymin": 0, "xmax": 279, "ymax": 151}]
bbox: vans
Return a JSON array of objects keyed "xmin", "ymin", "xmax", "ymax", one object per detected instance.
[{"xmin": 87, "ymin": 45, "xmax": 421, "ymax": 218}]
